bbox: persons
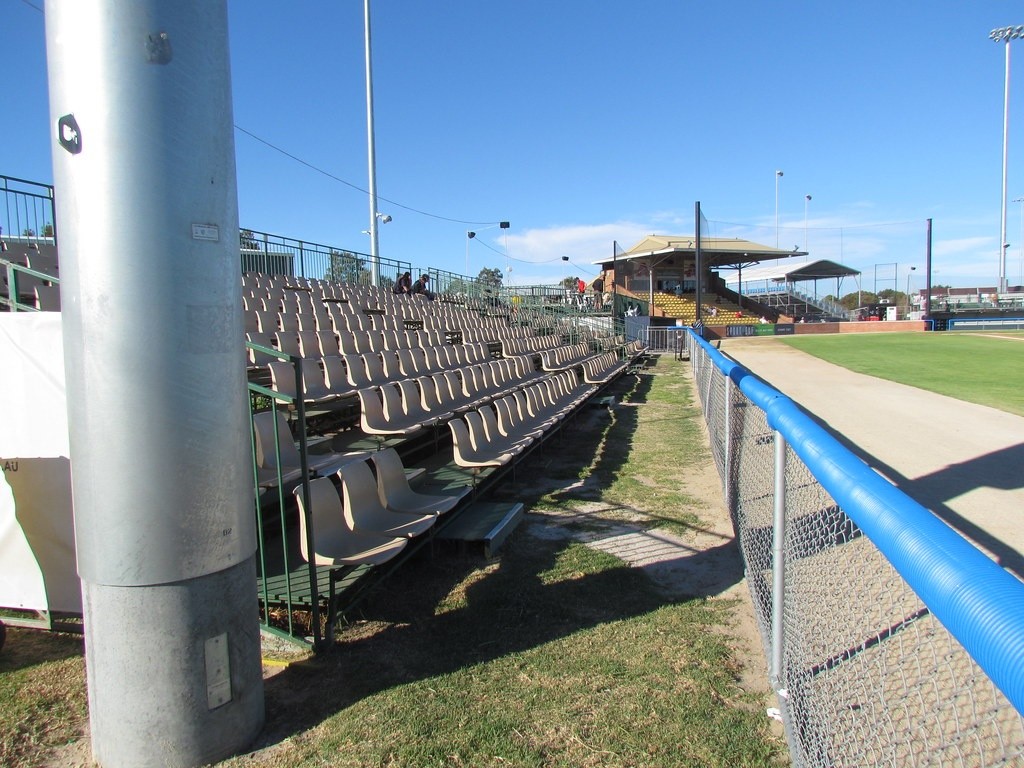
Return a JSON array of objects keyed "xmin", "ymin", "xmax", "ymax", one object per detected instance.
[
  {"xmin": 992, "ymin": 293, "xmax": 1000, "ymax": 308},
  {"xmin": 392, "ymin": 272, "xmax": 416, "ymax": 297},
  {"xmin": 944, "ymin": 300, "xmax": 951, "ymax": 312},
  {"xmin": 705, "ymin": 305, "xmax": 718, "ymax": 317},
  {"xmin": 574, "ymin": 277, "xmax": 589, "ymax": 310},
  {"xmin": 759, "ymin": 315, "xmax": 771, "ymax": 325},
  {"xmin": 604, "ymin": 282, "xmax": 615, "ymax": 308},
  {"xmin": 482, "ymin": 289, "xmax": 501, "ymax": 307},
  {"xmin": 592, "ymin": 275, "xmax": 606, "ymax": 310},
  {"xmin": 411, "ymin": 274, "xmax": 435, "ymax": 300},
  {"xmin": 734, "ymin": 310, "xmax": 744, "ymax": 319},
  {"xmin": 632, "ymin": 304, "xmax": 642, "ymax": 317},
  {"xmin": 794, "ymin": 314, "xmax": 806, "ymax": 324},
  {"xmin": 882, "ymin": 312, "xmax": 887, "ymax": 321}
]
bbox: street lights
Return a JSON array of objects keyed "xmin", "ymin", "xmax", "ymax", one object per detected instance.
[
  {"xmin": 1013, "ymin": 197, "xmax": 1024, "ymax": 287},
  {"xmin": 989, "ymin": 24, "xmax": 1024, "ymax": 293},
  {"xmin": 774, "ymin": 170, "xmax": 784, "ymax": 267},
  {"xmin": 803, "ymin": 194, "xmax": 811, "ymax": 315},
  {"xmin": 906, "ymin": 266, "xmax": 917, "ymax": 305}
]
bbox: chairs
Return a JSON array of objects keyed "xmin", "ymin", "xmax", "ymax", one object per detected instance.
[
  {"xmin": 0, "ymin": 237, "xmax": 632, "ymax": 568},
  {"xmin": 633, "ymin": 290, "xmax": 774, "ymax": 326}
]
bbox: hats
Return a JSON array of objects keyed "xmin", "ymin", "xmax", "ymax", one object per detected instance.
[
  {"xmin": 422, "ymin": 274, "xmax": 429, "ymax": 282},
  {"xmin": 575, "ymin": 277, "xmax": 579, "ymax": 279}
]
